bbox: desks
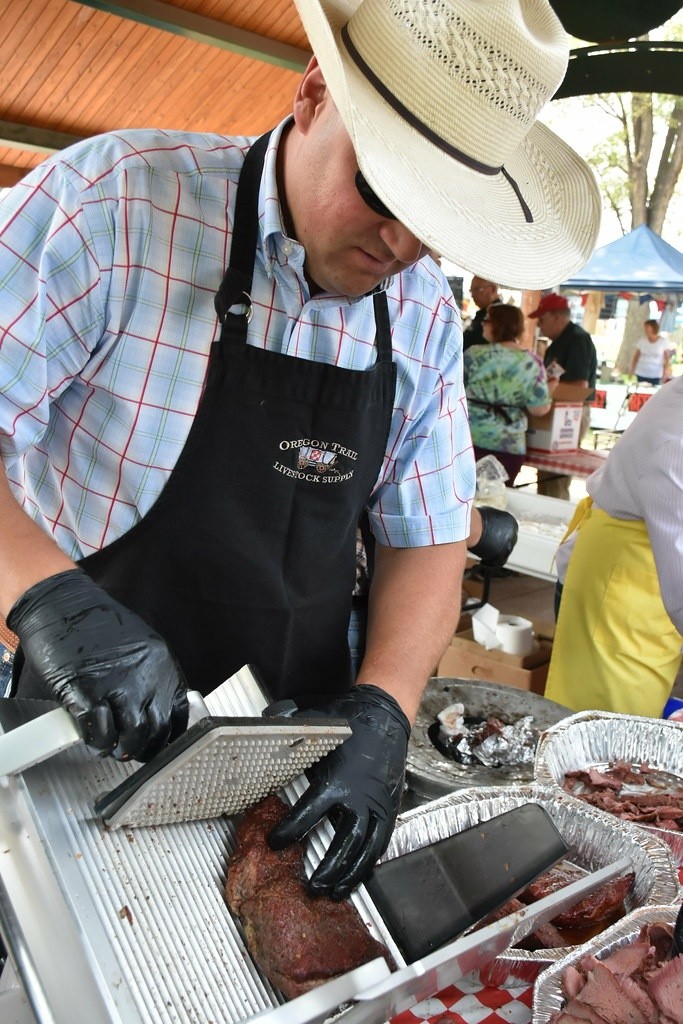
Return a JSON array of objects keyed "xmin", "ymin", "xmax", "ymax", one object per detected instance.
[{"xmin": 522, "ymin": 448, "xmax": 607, "ymax": 480}]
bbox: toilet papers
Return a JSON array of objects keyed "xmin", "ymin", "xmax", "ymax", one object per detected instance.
[{"xmin": 470, "ymin": 603, "xmax": 534, "ymax": 656}]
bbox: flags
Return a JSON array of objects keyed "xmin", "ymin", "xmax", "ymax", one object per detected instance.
[
  {"xmin": 581, "ymin": 293, "xmax": 587, "ymax": 306},
  {"xmin": 605, "ymin": 294, "xmax": 615, "ymax": 299},
  {"xmin": 640, "ymin": 294, "xmax": 651, "ymax": 305},
  {"xmin": 619, "ymin": 291, "xmax": 632, "ymax": 300},
  {"xmin": 657, "ymin": 301, "xmax": 666, "ymax": 310}
]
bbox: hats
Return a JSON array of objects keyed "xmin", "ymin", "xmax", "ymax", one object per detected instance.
[
  {"xmin": 528, "ymin": 292, "xmax": 568, "ymax": 319},
  {"xmin": 294, "ymin": 0, "xmax": 601, "ymax": 292}
]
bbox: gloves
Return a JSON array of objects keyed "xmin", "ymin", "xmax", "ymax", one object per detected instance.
[
  {"xmin": 4, "ymin": 568, "xmax": 189, "ymax": 764},
  {"xmin": 264, "ymin": 683, "xmax": 411, "ymax": 902}
]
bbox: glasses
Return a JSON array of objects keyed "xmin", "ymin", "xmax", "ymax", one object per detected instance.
[
  {"xmin": 468, "ymin": 286, "xmax": 496, "ymax": 294},
  {"xmin": 355, "ymin": 170, "xmax": 400, "ymax": 221}
]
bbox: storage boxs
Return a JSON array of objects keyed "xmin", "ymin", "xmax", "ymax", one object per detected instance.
[{"xmin": 435, "ymin": 624, "xmax": 554, "ymax": 697}]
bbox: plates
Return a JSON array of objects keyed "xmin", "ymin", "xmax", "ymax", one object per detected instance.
[{"xmin": 406, "ymin": 677, "xmax": 581, "ymax": 790}]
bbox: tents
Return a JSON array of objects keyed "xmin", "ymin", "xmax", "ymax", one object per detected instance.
[{"xmin": 558, "ymin": 223, "xmax": 683, "ymax": 295}]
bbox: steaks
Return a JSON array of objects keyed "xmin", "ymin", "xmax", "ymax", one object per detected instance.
[
  {"xmin": 226, "ymin": 792, "xmax": 309, "ymax": 912},
  {"xmin": 240, "ymin": 873, "xmax": 388, "ymax": 1000}
]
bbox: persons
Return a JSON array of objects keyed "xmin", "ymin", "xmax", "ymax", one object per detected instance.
[
  {"xmin": 528, "ymin": 295, "xmax": 597, "ymax": 501},
  {"xmin": 463, "ymin": 304, "xmax": 553, "ymax": 487},
  {"xmin": 0, "ymin": 0, "xmax": 602, "ymax": 898},
  {"xmin": 463, "ymin": 276, "xmax": 504, "ymax": 388},
  {"xmin": 546, "ymin": 374, "xmax": 683, "ymax": 719},
  {"xmin": 628, "ymin": 319, "xmax": 672, "ymax": 385}
]
